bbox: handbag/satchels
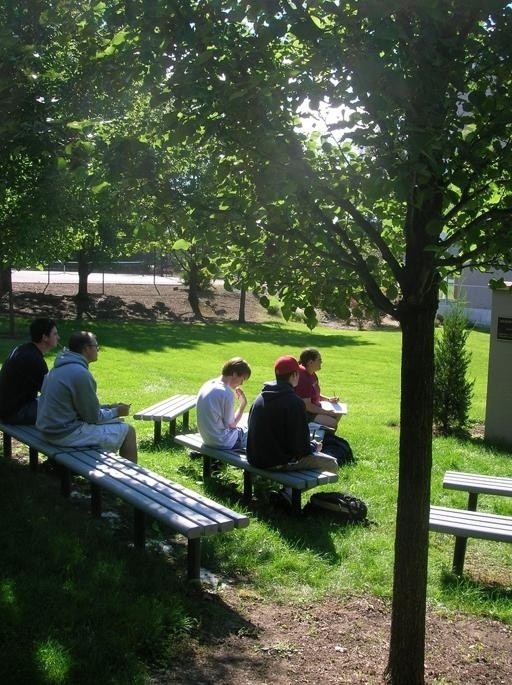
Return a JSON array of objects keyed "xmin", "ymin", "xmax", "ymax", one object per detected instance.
[{"xmin": 324, "ymin": 435, "xmax": 357, "ymax": 467}]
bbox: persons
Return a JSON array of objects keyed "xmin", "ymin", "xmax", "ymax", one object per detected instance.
[
  {"xmin": 35, "ymin": 328, "xmax": 140, "ymax": 471},
  {"xmin": 293, "ymin": 348, "xmax": 356, "ymax": 469},
  {"xmin": 243, "ymin": 354, "xmax": 341, "ymax": 515},
  {"xmin": 0, "ymin": 317, "xmax": 69, "ymax": 476},
  {"xmin": 193, "ymin": 357, "xmax": 257, "ymax": 454}
]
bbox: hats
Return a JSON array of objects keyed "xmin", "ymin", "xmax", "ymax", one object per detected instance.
[{"xmin": 275, "ymin": 355, "xmax": 307, "ymax": 376}]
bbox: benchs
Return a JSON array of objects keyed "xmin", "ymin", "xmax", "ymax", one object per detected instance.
[
  {"xmin": 135, "ymin": 394, "xmax": 200, "ymax": 446},
  {"xmin": 173, "ymin": 430, "xmax": 337, "ymax": 523},
  {"xmin": 0, "ymin": 425, "xmax": 250, "ymax": 595},
  {"xmin": 443, "ymin": 469, "xmax": 512, "ymax": 512},
  {"xmin": 42, "ymin": 259, "xmax": 147, "ymax": 274},
  {"xmin": 427, "ymin": 505, "xmax": 512, "ymax": 579}
]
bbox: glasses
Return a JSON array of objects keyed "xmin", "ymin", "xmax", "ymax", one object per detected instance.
[{"xmin": 87, "ymin": 343, "xmax": 101, "ymax": 352}]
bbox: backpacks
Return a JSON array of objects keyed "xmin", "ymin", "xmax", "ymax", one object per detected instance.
[{"xmin": 310, "ymin": 491, "xmax": 371, "ymax": 522}]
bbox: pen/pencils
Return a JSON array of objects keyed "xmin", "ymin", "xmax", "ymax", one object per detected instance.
[{"xmin": 312, "ymin": 429, "xmax": 317, "ymax": 440}]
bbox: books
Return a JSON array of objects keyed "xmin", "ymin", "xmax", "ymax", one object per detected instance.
[{"xmin": 317, "ymin": 398, "xmax": 348, "ymax": 417}]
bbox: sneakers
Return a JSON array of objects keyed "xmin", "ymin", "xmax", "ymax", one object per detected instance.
[{"xmin": 268, "ymin": 489, "xmax": 293, "ymax": 511}]
